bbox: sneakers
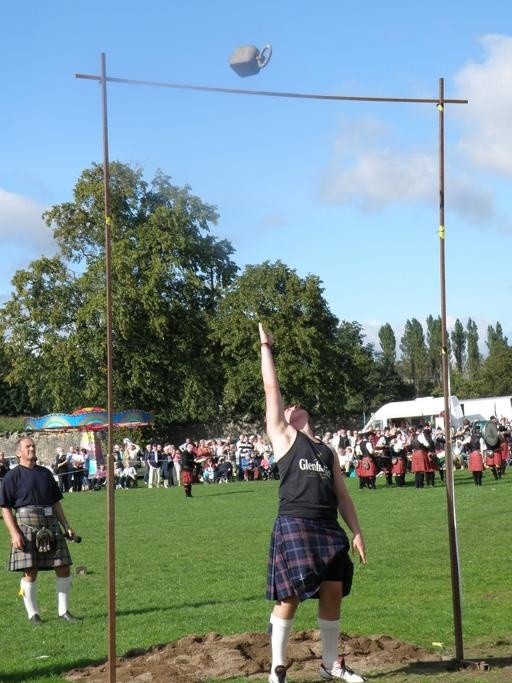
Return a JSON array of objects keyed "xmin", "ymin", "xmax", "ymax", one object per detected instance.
[
  {"xmin": 268, "ymin": 664, "xmax": 286, "ymax": 683},
  {"xmin": 319, "ymin": 656, "xmax": 365, "ymax": 683}
]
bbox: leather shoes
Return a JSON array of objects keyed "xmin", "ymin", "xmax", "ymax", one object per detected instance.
[
  {"xmin": 27, "ymin": 614, "xmax": 41, "ymax": 626},
  {"xmin": 55, "ymin": 611, "xmax": 80, "ymax": 622}
]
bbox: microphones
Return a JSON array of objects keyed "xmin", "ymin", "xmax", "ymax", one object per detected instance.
[{"xmin": 63, "ymin": 533, "xmax": 82, "ymax": 543}]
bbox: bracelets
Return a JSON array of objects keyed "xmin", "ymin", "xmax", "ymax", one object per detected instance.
[{"xmin": 259, "ymin": 341, "xmax": 273, "ymax": 349}]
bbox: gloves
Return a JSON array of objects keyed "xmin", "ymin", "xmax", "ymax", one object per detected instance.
[{"xmin": 34, "ymin": 526, "xmax": 54, "ymax": 553}]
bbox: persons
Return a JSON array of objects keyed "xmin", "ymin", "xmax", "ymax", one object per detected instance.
[
  {"xmin": 0, "ymin": 437, "xmax": 83, "ymax": 625},
  {"xmin": 257, "ymin": 322, "xmax": 370, "ymax": 683},
  {"xmin": 0, "ymin": 452, "xmax": 12, "ymax": 519}
]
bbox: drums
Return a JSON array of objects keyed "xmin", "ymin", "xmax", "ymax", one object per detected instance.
[
  {"xmin": 473, "ymin": 421, "xmax": 498, "ymax": 446},
  {"xmin": 437, "ymin": 450, "xmax": 459, "ymax": 468}
]
